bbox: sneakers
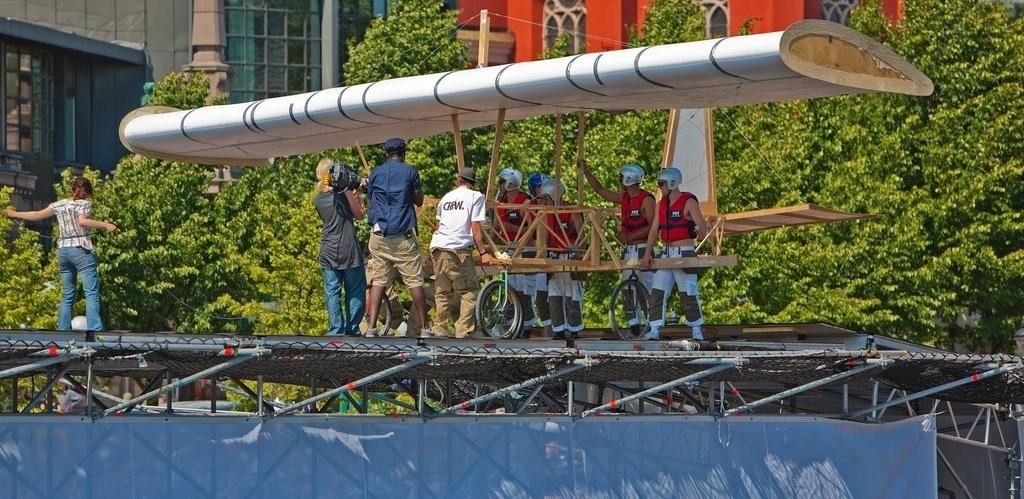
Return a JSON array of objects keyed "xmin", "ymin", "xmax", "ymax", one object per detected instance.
[
  {"xmin": 571, "ymin": 331, "xmax": 579, "ymax": 340},
  {"xmin": 647, "ymin": 326, "xmax": 661, "ymax": 340},
  {"xmin": 551, "ymin": 331, "xmax": 567, "ymax": 340},
  {"xmin": 364, "ymin": 326, "xmax": 381, "ymax": 338},
  {"xmin": 692, "ymin": 326, "xmax": 704, "ymax": 340},
  {"xmin": 420, "ymin": 327, "xmax": 435, "ymax": 338}
]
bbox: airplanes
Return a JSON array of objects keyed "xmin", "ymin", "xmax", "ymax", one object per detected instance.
[{"xmin": 117, "ymin": 6, "xmax": 935, "ymax": 339}]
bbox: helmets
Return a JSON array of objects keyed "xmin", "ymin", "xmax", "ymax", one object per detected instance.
[
  {"xmin": 499, "ymin": 167, "xmax": 523, "ymax": 191},
  {"xmin": 539, "ymin": 179, "xmax": 566, "ymax": 201},
  {"xmin": 527, "ymin": 172, "xmax": 548, "ymax": 196},
  {"xmin": 657, "ymin": 167, "xmax": 683, "ymax": 191},
  {"xmin": 619, "ymin": 164, "xmax": 644, "ymax": 186}
]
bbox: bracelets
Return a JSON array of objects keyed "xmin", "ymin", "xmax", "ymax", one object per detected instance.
[{"xmin": 480, "ymin": 250, "xmax": 487, "ymax": 256}]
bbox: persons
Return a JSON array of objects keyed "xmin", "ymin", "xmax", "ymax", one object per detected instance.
[
  {"xmin": 358, "ymin": 240, "xmax": 403, "ymax": 337},
  {"xmin": 640, "ymin": 168, "xmax": 707, "ymax": 341},
  {"xmin": 491, "ymin": 169, "xmax": 588, "ymax": 340},
  {"xmin": 429, "ymin": 168, "xmax": 493, "ymax": 338},
  {"xmin": 366, "ymin": 138, "xmax": 435, "ymax": 338},
  {"xmin": 2, "ymin": 177, "xmax": 117, "ymax": 332},
  {"xmin": 406, "ymin": 257, "xmax": 461, "ymax": 338},
  {"xmin": 312, "ymin": 158, "xmax": 367, "ymax": 337},
  {"xmin": 576, "ymin": 159, "xmax": 656, "ymax": 340},
  {"xmin": 58, "ymin": 378, "xmax": 83, "ymax": 413}
]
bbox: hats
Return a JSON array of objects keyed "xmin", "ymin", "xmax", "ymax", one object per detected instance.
[
  {"xmin": 384, "ymin": 137, "xmax": 406, "ymax": 153},
  {"xmin": 459, "ymin": 167, "xmax": 478, "ymax": 182}
]
